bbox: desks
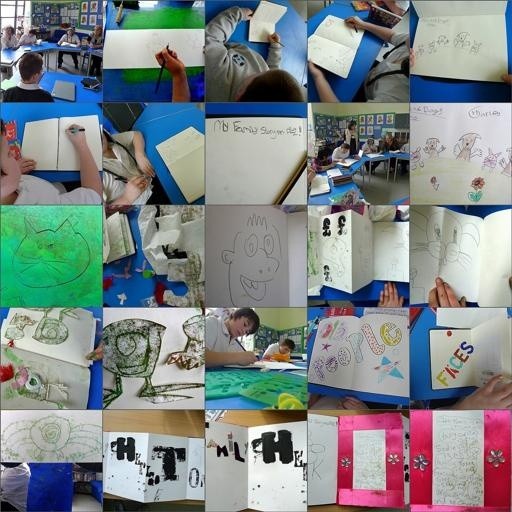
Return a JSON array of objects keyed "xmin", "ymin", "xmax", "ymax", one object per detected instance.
[
  {"xmin": 307, "ymin": 149, "xmax": 410, "ymax": 205},
  {"xmin": 307, "ymin": 4, "xmax": 390, "ymax": 103},
  {"xmin": 205, "ymin": 0, "xmax": 307, "ymax": 90},
  {"xmin": 0, "ymin": 42, "xmax": 104, "ymax": 105},
  {"xmin": 409, "ymin": 1, "xmax": 512, "ymax": 103}
]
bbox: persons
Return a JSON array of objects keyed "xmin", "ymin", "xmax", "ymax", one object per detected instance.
[
  {"xmin": 377, "ymin": 282, "xmax": 404, "ymax": 308},
  {"xmin": 450, "ymin": 373, "xmax": 512, "ymax": 409},
  {"xmin": 205, "ymin": 5, "xmax": 305, "ymax": 102},
  {"xmin": 154, "ymin": 48, "xmax": 192, "ymax": 103},
  {"xmin": 410, "ymin": 48, "xmax": 512, "ymax": 85},
  {"xmin": 380, "ymin": 1, "xmax": 410, "ymax": 17},
  {"xmin": 0, "ymin": 462, "xmax": 30, "ymax": 511},
  {"xmin": 308, "ymin": 120, "xmax": 410, "ymax": 189},
  {"xmin": 309, "ymin": 15, "xmax": 410, "ymax": 103},
  {"xmin": 1, "ymin": 118, "xmax": 170, "ymax": 205},
  {"xmin": 336, "ymin": 396, "xmax": 369, "ymax": 410},
  {"xmin": 205, "ymin": 308, "xmax": 296, "ymax": 369},
  {"xmin": 1, "ymin": 25, "xmax": 102, "ymax": 102},
  {"xmin": 429, "ymin": 277, "xmax": 466, "ymax": 307}
]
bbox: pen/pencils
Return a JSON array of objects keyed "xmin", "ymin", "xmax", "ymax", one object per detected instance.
[
  {"xmin": 272, "ymin": 38, "xmax": 285, "ymax": 47},
  {"xmin": 352, "ymin": 23, "xmax": 358, "ymax": 33},
  {"xmin": 115, "ymin": 1, "xmax": 124, "ymax": 26},
  {"xmin": 69, "ymin": 128, "xmax": 85, "ymax": 133},
  {"xmin": 235, "ymin": 338, "xmax": 247, "ymax": 351},
  {"xmin": 155, "ymin": 42, "xmax": 169, "ymax": 94}
]
